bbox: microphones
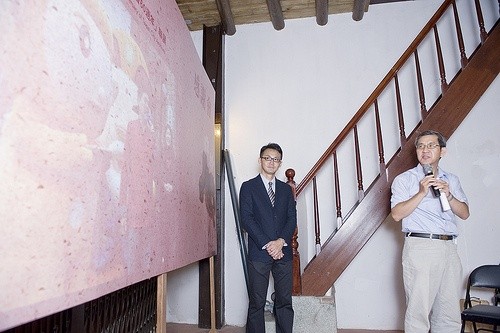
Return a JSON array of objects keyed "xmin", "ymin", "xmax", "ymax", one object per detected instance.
[{"xmin": 423, "ymin": 163, "xmax": 441, "ymax": 197}]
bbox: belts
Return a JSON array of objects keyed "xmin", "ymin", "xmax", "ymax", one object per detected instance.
[{"xmin": 406, "ymin": 232, "xmax": 457, "ymax": 240}]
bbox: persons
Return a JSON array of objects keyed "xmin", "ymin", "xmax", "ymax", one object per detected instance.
[
  {"xmin": 240, "ymin": 143, "xmax": 297, "ymax": 333},
  {"xmin": 391, "ymin": 130, "xmax": 471, "ymax": 333}
]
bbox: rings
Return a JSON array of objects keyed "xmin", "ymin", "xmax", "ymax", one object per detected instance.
[{"xmin": 270, "ymin": 249, "xmax": 272, "ymax": 252}]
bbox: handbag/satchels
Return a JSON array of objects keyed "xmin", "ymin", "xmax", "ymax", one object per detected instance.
[{"xmin": 460, "ymin": 297, "xmax": 490, "ymax": 333}]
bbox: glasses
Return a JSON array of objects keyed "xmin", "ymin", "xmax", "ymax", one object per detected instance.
[
  {"xmin": 261, "ymin": 157, "xmax": 280, "ymax": 162},
  {"xmin": 416, "ymin": 143, "xmax": 441, "ymax": 149}
]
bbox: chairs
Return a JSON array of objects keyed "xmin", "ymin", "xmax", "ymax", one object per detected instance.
[{"xmin": 460, "ymin": 263, "xmax": 500, "ymax": 333}]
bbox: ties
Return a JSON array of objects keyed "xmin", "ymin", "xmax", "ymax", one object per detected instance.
[{"xmin": 267, "ymin": 182, "xmax": 276, "ymax": 208}]
